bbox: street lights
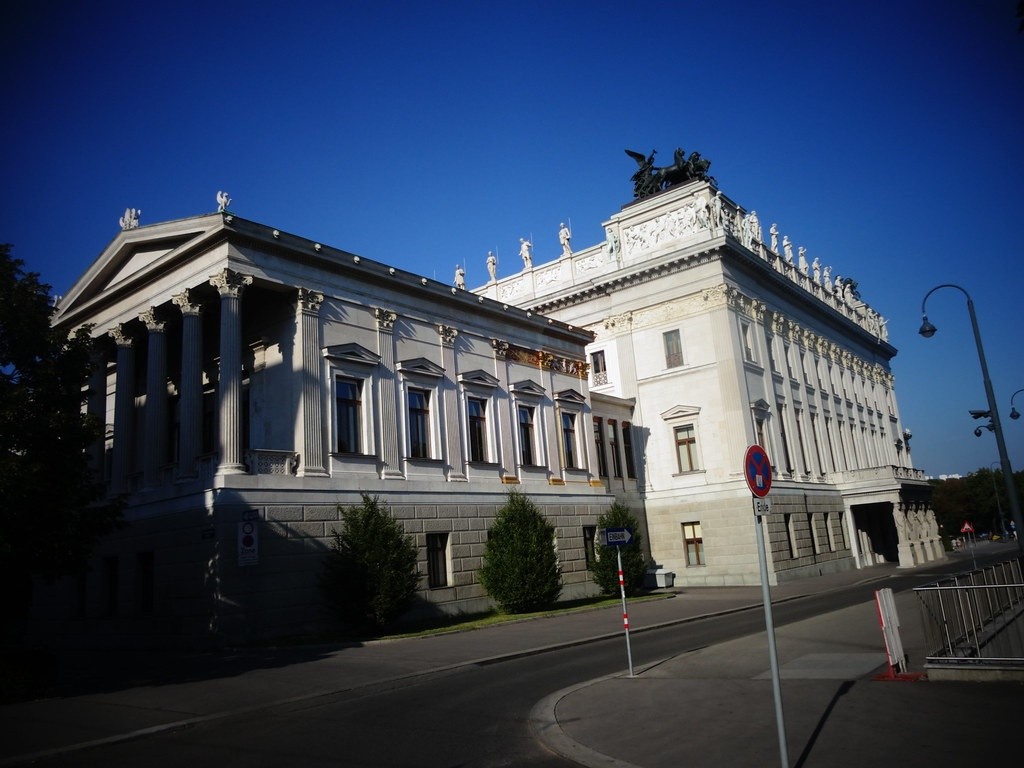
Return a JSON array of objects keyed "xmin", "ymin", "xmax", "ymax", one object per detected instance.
[{"xmin": 918, "ymin": 283, "xmax": 1023, "ymax": 578}]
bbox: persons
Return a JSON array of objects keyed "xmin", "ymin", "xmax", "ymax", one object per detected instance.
[
  {"xmin": 711, "ymin": 190, "xmax": 726, "ymax": 228},
  {"xmin": 453, "ymin": 264, "xmax": 464, "ymax": 289},
  {"xmin": 559, "ymin": 223, "xmax": 573, "ymax": 254},
  {"xmin": 741, "ymin": 211, "xmax": 890, "ymax": 343},
  {"xmin": 518, "ymin": 238, "xmax": 532, "ymax": 267},
  {"xmin": 486, "ymin": 251, "xmax": 496, "ymax": 280},
  {"xmin": 1010, "ymin": 521, "xmax": 1018, "ymax": 542},
  {"xmin": 950, "ymin": 537, "xmax": 968, "ymax": 551},
  {"xmin": 988, "ymin": 532, "xmax": 994, "ymax": 541}
]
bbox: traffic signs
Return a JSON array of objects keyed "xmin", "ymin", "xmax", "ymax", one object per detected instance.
[{"xmin": 604, "ymin": 526, "xmax": 634, "ymax": 547}]
